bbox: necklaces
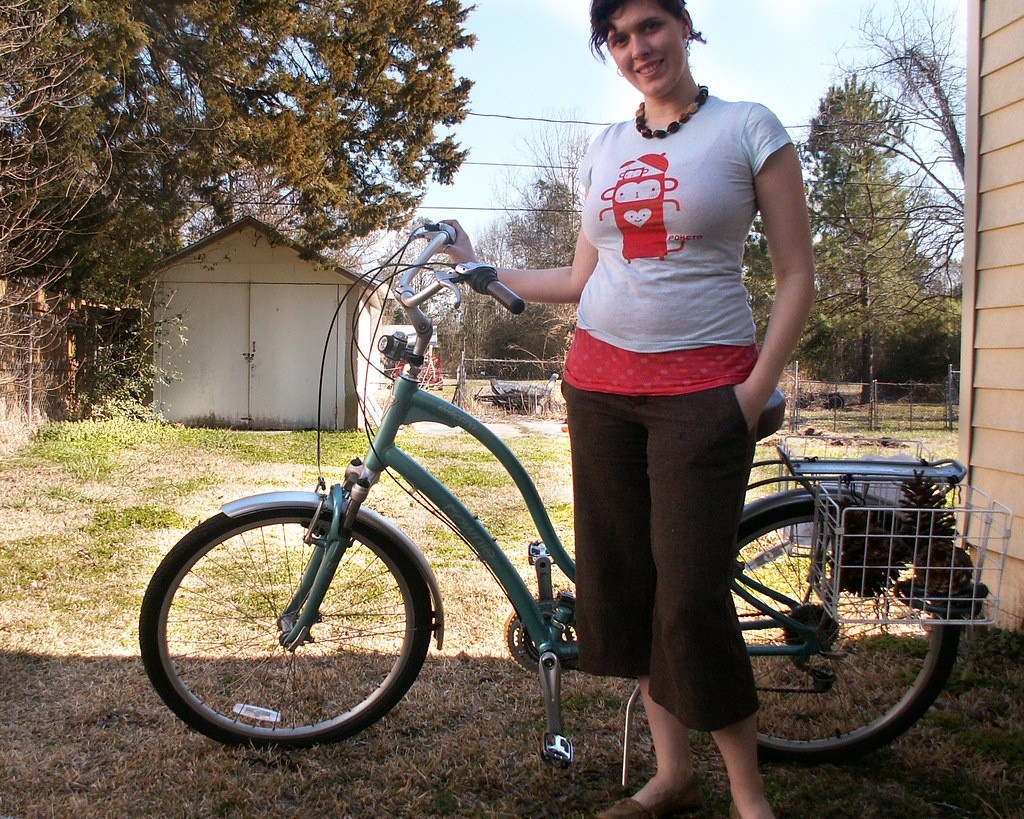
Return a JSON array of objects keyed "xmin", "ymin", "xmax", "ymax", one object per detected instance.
[{"xmin": 635, "ymin": 85, "xmax": 709, "ymax": 140}]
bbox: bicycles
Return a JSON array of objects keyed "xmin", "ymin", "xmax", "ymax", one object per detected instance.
[{"xmin": 137, "ymin": 210, "xmax": 1015, "ymax": 779}]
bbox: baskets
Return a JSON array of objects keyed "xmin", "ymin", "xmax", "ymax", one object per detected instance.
[{"xmin": 776, "ymin": 436, "xmax": 1013, "ymax": 626}]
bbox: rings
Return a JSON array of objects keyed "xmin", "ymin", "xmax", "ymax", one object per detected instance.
[{"xmin": 442, "ymin": 244, "xmax": 451, "ymax": 254}]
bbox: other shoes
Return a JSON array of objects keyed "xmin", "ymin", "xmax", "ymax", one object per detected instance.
[
  {"xmin": 597, "ymin": 772, "xmax": 703, "ymax": 819},
  {"xmin": 729, "ymin": 798, "xmax": 779, "ymax": 819}
]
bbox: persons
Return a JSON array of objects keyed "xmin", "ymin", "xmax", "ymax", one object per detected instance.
[{"xmin": 426, "ymin": 0, "xmax": 813, "ymax": 819}]
